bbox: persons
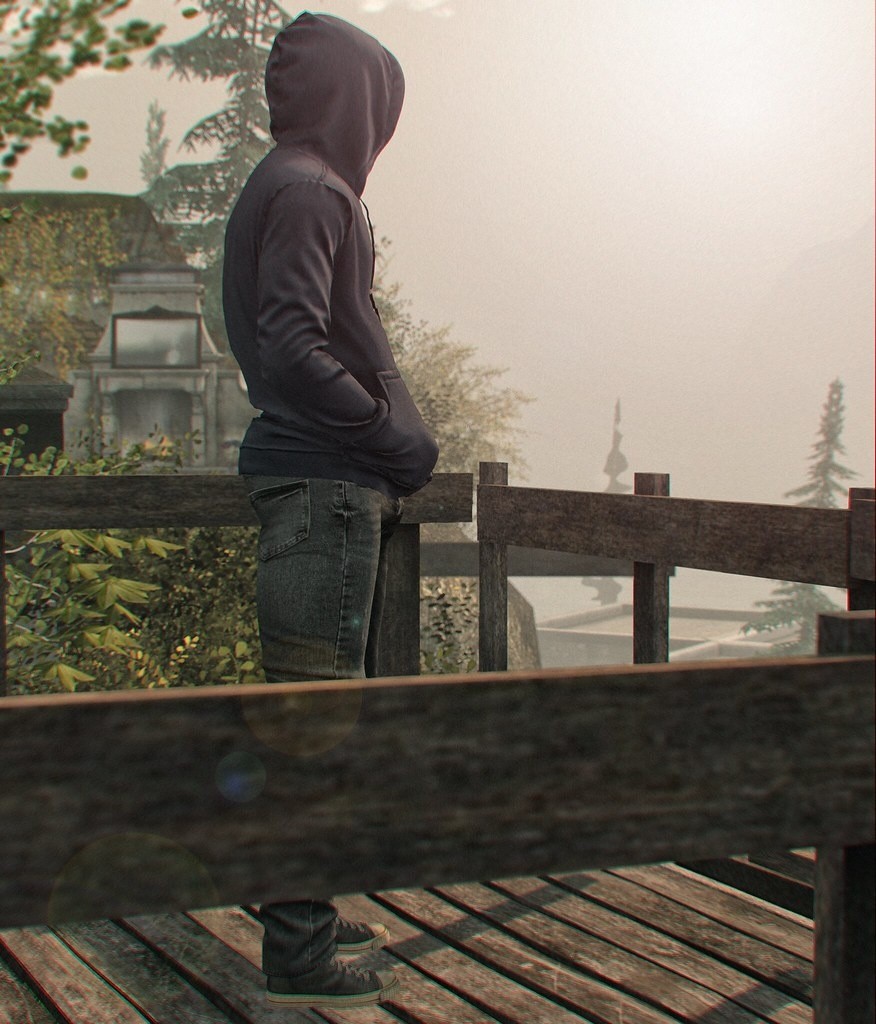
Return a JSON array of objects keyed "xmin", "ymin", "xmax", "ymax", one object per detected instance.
[{"xmin": 221, "ymin": 9, "xmax": 439, "ymax": 1003}]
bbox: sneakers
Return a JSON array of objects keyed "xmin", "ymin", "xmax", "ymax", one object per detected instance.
[
  {"xmin": 267, "ymin": 957, "xmax": 400, "ymax": 1008},
  {"xmin": 336, "ymin": 915, "xmax": 391, "ymax": 955}
]
